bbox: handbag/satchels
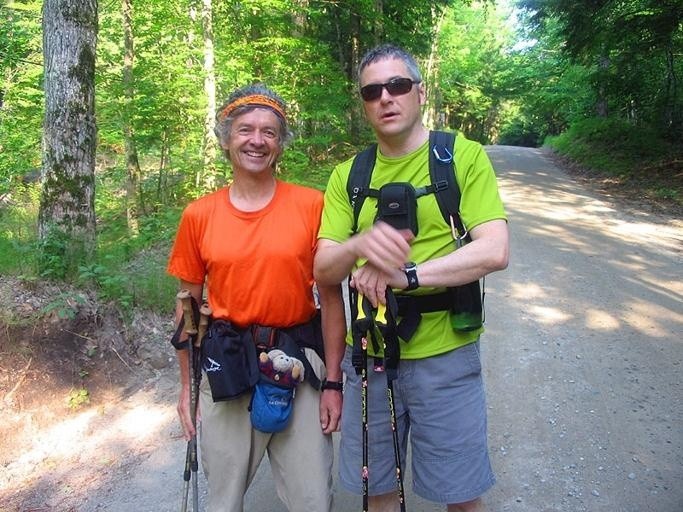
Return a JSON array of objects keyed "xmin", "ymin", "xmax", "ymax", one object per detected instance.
[
  {"xmin": 399, "ymin": 261, "xmax": 418, "ymax": 292},
  {"xmin": 318, "ymin": 377, "xmax": 348, "ymax": 394}
]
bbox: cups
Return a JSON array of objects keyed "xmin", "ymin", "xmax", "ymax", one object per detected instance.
[{"xmin": 359, "ymin": 77, "xmax": 421, "ymax": 103}]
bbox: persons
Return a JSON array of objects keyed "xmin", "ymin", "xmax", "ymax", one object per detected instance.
[
  {"xmin": 311, "ymin": 44, "xmax": 510, "ymax": 511},
  {"xmin": 169, "ymin": 83, "xmax": 347, "ymax": 511}
]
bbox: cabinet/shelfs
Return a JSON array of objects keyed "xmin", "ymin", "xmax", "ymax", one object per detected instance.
[{"xmin": 447, "ymin": 278, "xmax": 483, "ymax": 335}]
